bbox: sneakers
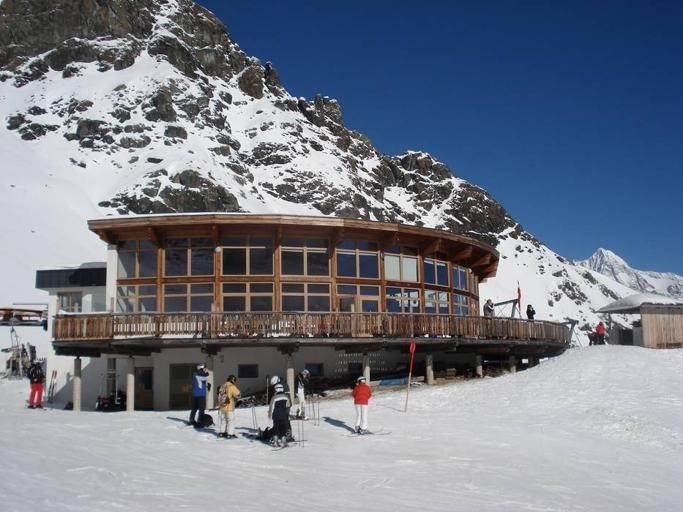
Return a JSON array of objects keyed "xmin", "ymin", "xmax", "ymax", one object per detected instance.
[
  {"xmin": 28, "ymin": 405, "xmax": 44, "ymax": 409},
  {"xmin": 219, "ymin": 432, "xmax": 234, "ymax": 439},
  {"xmin": 355, "ymin": 428, "xmax": 368, "ymax": 434},
  {"xmin": 295, "ymin": 414, "xmax": 309, "ymax": 420}
]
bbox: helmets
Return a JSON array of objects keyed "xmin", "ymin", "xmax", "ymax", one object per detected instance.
[
  {"xmin": 301, "ymin": 369, "xmax": 310, "ymax": 375},
  {"xmin": 357, "ymin": 376, "xmax": 366, "ymax": 383},
  {"xmin": 274, "ymin": 383, "xmax": 285, "ymax": 393},
  {"xmin": 270, "ymin": 375, "xmax": 281, "ymax": 386},
  {"xmin": 196, "ymin": 363, "xmax": 206, "ymax": 372},
  {"xmin": 227, "ymin": 375, "xmax": 237, "ymax": 384}
]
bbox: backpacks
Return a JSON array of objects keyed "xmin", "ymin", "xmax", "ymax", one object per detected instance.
[{"xmin": 217, "ymin": 384, "xmax": 233, "ymax": 406}]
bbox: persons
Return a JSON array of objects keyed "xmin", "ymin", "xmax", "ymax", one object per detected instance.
[
  {"xmin": 27, "ymin": 358, "xmax": 47, "ymax": 409},
  {"xmin": 483, "ymin": 299, "xmax": 494, "ymax": 316},
  {"xmin": 295, "ymin": 370, "xmax": 310, "ymax": 418},
  {"xmin": 189, "ymin": 363, "xmax": 209, "ymax": 429},
  {"xmin": 353, "ymin": 376, "xmax": 371, "ymax": 433},
  {"xmin": 217, "ymin": 375, "xmax": 241, "ymax": 438},
  {"xmin": 526, "ymin": 304, "xmax": 535, "ymax": 319},
  {"xmin": 268, "ymin": 376, "xmax": 295, "ymax": 446},
  {"xmin": 589, "ymin": 322, "xmax": 606, "ymax": 345}
]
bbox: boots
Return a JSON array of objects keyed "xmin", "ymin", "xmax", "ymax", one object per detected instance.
[{"xmin": 273, "ymin": 435, "xmax": 288, "ymax": 447}]
